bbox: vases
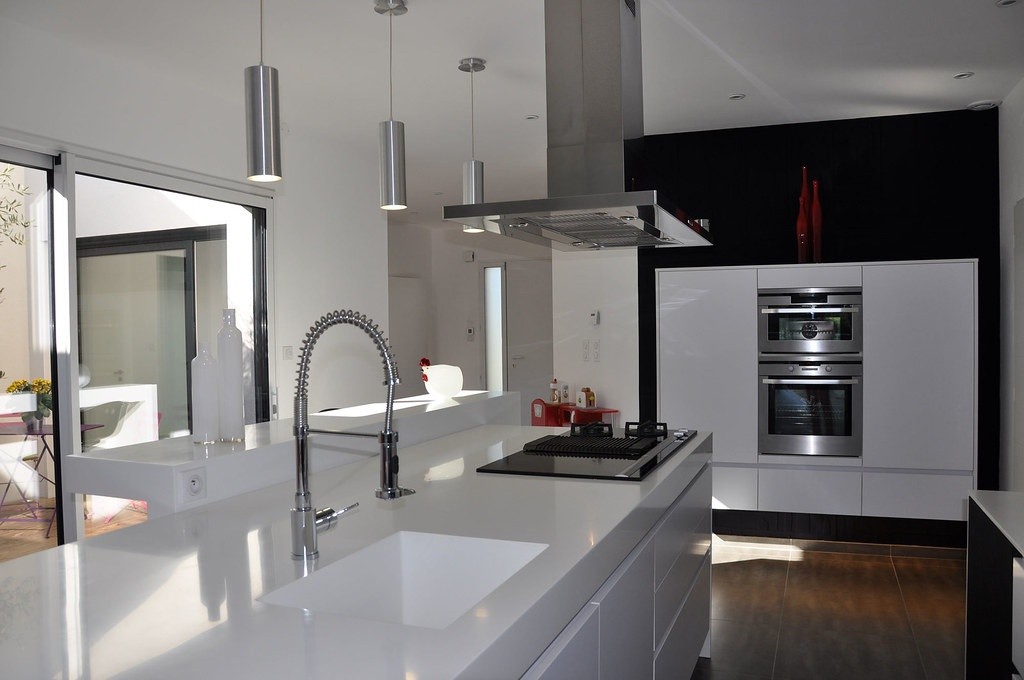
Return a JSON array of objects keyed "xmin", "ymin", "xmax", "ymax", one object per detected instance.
[
  {"xmin": 192, "ymin": 343, "xmax": 219, "ymax": 444},
  {"xmin": 216, "ymin": 309, "xmax": 245, "ymax": 442},
  {"xmin": 24, "ymin": 415, "xmax": 42, "ymax": 431}
]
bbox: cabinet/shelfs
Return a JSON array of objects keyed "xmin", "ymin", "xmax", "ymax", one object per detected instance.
[{"xmin": 520, "ymin": 463, "xmax": 713, "ymax": 679}]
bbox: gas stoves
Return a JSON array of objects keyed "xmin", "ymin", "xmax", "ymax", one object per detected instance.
[{"xmin": 476, "ymin": 420, "xmax": 700, "ymax": 483}]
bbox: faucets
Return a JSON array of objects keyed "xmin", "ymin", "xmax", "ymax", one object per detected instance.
[{"xmin": 291, "ymin": 310, "xmax": 402, "ymax": 561}]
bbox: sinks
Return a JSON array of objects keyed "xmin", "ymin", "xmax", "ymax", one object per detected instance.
[{"xmin": 253, "ymin": 528, "xmax": 551, "ymax": 629}]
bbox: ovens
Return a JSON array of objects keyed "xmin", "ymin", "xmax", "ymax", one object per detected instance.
[{"xmin": 756, "ymin": 286, "xmax": 865, "ymax": 457}]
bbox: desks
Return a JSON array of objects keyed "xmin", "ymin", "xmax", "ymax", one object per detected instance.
[{"xmin": 0, "ymin": 421, "xmax": 104, "ymax": 538}]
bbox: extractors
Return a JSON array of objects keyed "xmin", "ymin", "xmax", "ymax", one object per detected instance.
[{"xmin": 445, "ymin": 177, "xmax": 715, "ymax": 254}]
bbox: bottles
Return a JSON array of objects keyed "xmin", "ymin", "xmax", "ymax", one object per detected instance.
[
  {"xmin": 217, "ymin": 309, "xmax": 245, "ymax": 442},
  {"xmin": 191, "ymin": 340, "xmax": 220, "ymax": 445}
]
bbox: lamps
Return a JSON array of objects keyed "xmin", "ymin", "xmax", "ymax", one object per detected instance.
[
  {"xmin": 373, "ymin": 1, "xmax": 408, "ymax": 210},
  {"xmin": 243, "ymin": 2, "xmax": 282, "ymax": 184},
  {"xmin": 457, "ymin": 57, "xmax": 487, "ymax": 234}
]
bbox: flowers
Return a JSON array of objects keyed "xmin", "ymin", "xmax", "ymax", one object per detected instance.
[{"xmin": 7, "ymin": 377, "xmax": 54, "ymax": 422}]
